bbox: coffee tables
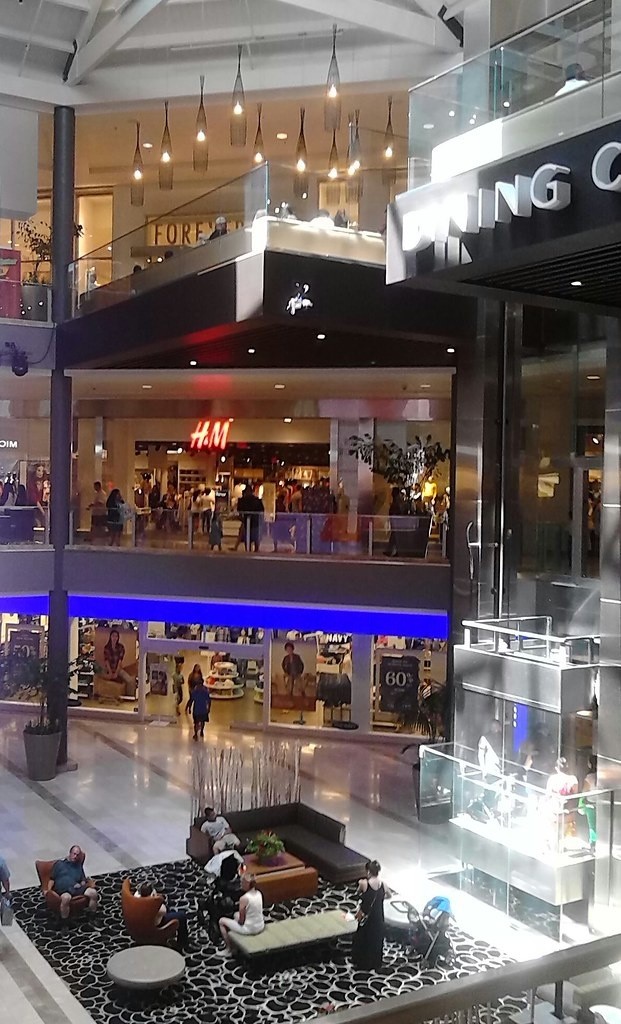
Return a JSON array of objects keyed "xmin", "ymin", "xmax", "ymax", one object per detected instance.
[{"xmin": 238, "ymin": 851, "xmax": 305, "ymax": 875}]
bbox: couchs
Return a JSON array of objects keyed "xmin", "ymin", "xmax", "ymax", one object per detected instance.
[{"xmin": 185, "ymin": 802, "xmax": 371, "ymax": 902}]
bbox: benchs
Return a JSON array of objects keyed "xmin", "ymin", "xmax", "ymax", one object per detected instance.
[{"xmin": 230, "ymin": 910, "xmax": 359, "ymax": 968}]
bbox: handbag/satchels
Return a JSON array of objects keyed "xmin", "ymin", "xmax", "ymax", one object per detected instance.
[
  {"xmin": 1, "ymin": 898, "xmax": 14, "ymax": 926},
  {"xmin": 345, "ymin": 908, "xmax": 355, "ymax": 922},
  {"xmin": 355, "ymin": 904, "xmax": 361, "ymax": 913},
  {"xmin": 356, "ymin": 909, "xmax": 370, "ymax": 927}
]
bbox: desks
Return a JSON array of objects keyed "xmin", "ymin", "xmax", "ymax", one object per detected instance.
[
  {"xmin": 383, "ymin": 898, "xmax": 436, "ymax": 945},
  {"xmin": 107, "ymin": 945, "xmax": 186, "ymax": 990}
]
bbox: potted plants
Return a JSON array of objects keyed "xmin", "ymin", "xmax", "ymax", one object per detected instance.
[
  {"xmin": 349, "ymin": 433, "xmax": 450, "ymax": 558},
  {"xmin": 16, "ymin": 219, "xmax": 84, "ymax": 321},
  {"xmin": 0, "ymin": 652, "xmax": 91, "ymax": 781}
]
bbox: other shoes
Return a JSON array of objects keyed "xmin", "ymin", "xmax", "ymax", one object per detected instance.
[
  {"xmin": 216, "ymin": 948, "xmax": 232, "ymax": 958},
  {"xmin": 232, "ymin": 947, "xmax": 238, "ymax": 955},
  {"xmin": 180, "ymin": 944, "xmax": 201, "ymax": 953},
  {"xmin": 89, "ymin": 919, "xmax": 104, "ymax": 928},
  {"xmin": 62, "ymin": 925, "xmax": 69, "ymax": 936}
]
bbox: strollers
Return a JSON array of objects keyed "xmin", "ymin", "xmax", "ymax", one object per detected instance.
[{"xmin": 390, "ymin": 897, "xmax": 457, "ymax": 971}]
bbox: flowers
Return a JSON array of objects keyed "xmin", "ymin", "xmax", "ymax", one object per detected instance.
[{"xmin": 244, "ymin": 829, "xmax": 285, "ymax": 864}]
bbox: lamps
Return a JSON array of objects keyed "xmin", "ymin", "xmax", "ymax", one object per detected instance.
[{"xmin": 129, "ymin": 23, "xmax": 396, "ymax": 206}]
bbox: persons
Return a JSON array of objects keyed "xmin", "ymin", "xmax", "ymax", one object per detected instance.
[
  {"xmin": 209, "ymin": 216, "xmax": 228, "ymax": 239},
  {"xmin": 351, "ymin": 860, "xmax": 392, "ymax": 969},
  {"xmin": 104, "ymin": 488, "xmax": 125, "ymax": 547},
  {"xmin": 201, "ymin": 807, "xmax": 242, "ymax": 855},
  {"xmin": 384, "ymin": 484, "xmax": 422, "ymax": 557},
  {"xmin": 209, "ymin": 511, "xmax": 223, "ymax": 551},
  {"xmin": 184, "ymin": 678, "xmax": 211, "ymax": 739},
  {"xmin": 134, "ymin": 883, "xmax": 188, "ymax": 939},
  {"xmin": 173, "ymin": 663, "xmax": 202, "ymax": 714},
  {"xmin": 83, "ymin": 481, "xmax": 108, "ymax": 542},
  {"xmin": 26, "ymin": 461, "xmax": 47, "ymax": 514},
  {"xmin": 47, "ymin": 846, "xmax": 97, "ymax": 928},
  {"xmin": 226, "ymin": 478, "xmax": 331, "ymax": 554},
  {"xmin": 0, "ymin": 856, "xmax": 10, "ymax": 893},
  {"xmin": 89, "ymin": 273, "xmax": 98, "ymax": 290},
  {"xmin": 99, "ymin": 630, "xmax": 134, "ymax": 696},
  {"xmin": 171, "ymin": 623, "xmax": 200, "ymax": 640},
  {"xmin": 545, "ymin": 755, "xmax": 598, "ymax": 850},
  {"xmin": 217, "ymin": 871, "xmax": 265, "ymax": 956},
  {"xmin": 477, "ymin": 720, "xmax": 507, "ymax": 781},
  {"xmin": 149, "ymin": 482, "xmax": 215, "ymax": 536},
  {"xmin": 282, "ymin": 642, "xmax": 305, "ymax": 696}
]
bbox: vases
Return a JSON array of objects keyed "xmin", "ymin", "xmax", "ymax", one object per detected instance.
[{"xmin": 265, "ymin": 850, "xmax": 283, "ymax": 864}]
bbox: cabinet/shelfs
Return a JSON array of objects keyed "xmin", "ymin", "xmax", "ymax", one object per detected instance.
[
  {"xmin": 204, "ymin": 670, "xmax": 244, "ymax": 699},
  {"xmin": 177, "ymin": 468, "xmax": 207, "ymax": 500}
]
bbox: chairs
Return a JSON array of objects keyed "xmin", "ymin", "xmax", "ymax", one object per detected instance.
[
  {"xmin": 122, "ymin": 878, "xmax": 179, "ymax": 946},
  {"xmin": 35, "ymin": 852, "xmax": 96, "ymax": 914}
]
